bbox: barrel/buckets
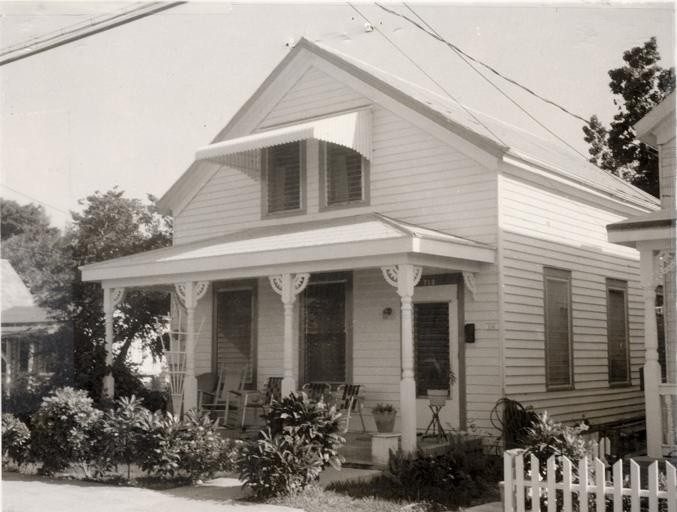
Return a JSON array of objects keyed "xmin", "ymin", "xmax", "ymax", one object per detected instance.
[
  {"xmin": 426, "ymin": 389, "xmax": 449, "ymax": 406},
  {"xmin": 371, "ymin": 412, "xmax": 397, "ymax": 433}
]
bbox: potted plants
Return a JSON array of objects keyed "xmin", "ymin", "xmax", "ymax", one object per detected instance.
[
  {"xmin": 412, "ymin": 356, "xmax": 457, "ymax": 406},
  {"xmin": 370, "ymin": 401, "xmax": 400, "ymax": 432}
]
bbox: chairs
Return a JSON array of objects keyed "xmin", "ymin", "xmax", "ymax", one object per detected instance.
[{"xmin": 194, "ymin": 362, "xmax": 367, "ymax": 436}]
